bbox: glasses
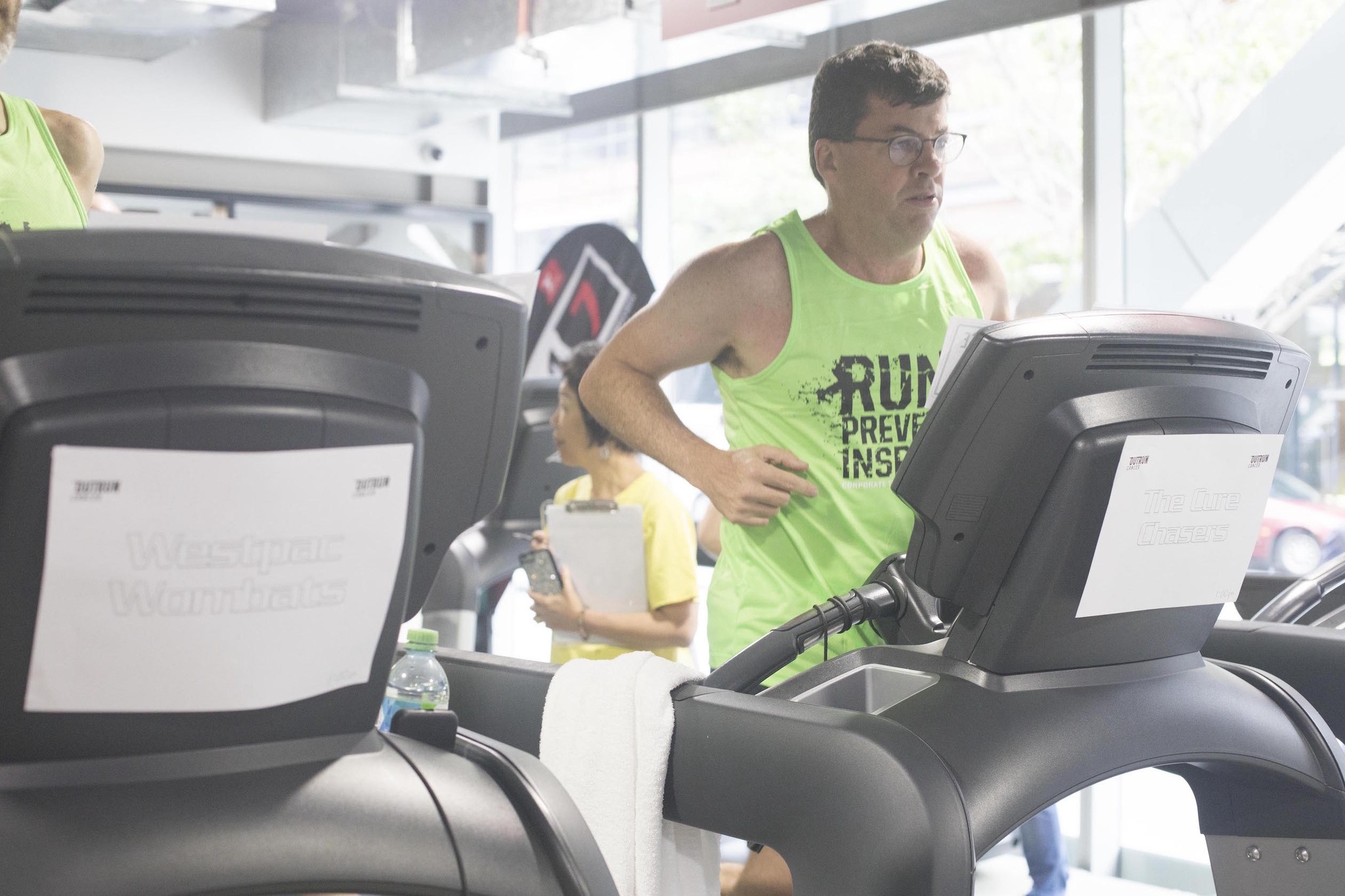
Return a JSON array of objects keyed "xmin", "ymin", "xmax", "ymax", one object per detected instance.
[{"xmin": 825, "ymin": 134, "xmax": 967, "ymax": 169}]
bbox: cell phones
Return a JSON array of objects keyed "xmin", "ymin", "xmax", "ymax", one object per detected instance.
[{"xmin": 519, "ymin": 548, "xmax": 561, "ymax": 595}]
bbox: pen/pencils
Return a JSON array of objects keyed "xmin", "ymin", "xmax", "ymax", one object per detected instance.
[{"xmin": 512, "ymin": 532, "xmax": 542, "ymax": 543}]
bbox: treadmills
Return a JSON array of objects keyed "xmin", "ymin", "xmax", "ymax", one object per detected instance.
[
  {"xmin": 0, "ymin": 232, "xmax": 624, "ymax": 894},
  {"xmin": 391, "ymin": 309, "xmax": 1345, "ymax": 896}
]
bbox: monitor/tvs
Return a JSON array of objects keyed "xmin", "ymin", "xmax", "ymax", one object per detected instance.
[
  {"xmin": 890, "ymin": 312, "xmax": 1306, "ymax": 673},
  {"xmin": 1, "ymin": 226, "xmax": 532, "ymax": 762}
]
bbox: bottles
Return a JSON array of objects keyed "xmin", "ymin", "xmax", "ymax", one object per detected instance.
[{"xmin": 376, "ymin": 628, "xmax": 448, "ymax": 734}]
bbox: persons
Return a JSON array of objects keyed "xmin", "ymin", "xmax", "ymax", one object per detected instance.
[
  {"xmin": 579, "ymin": 40, "xmax": 1009, "ymax": 895},
  {"xmin": 0, "ymin": 0, "xmax": 104, "ymax": 231},
  {"xmin": 529, "ymin": 339, "xmax": 696, "ymax": 663}
]
